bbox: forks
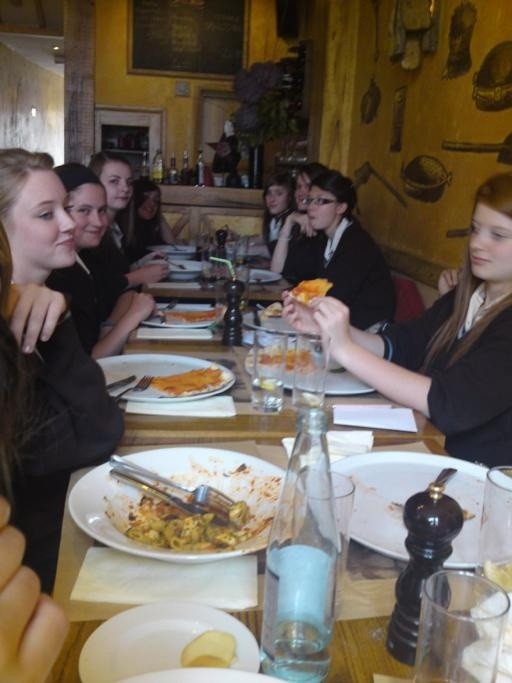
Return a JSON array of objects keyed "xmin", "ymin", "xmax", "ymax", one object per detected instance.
[
  {"xmin": 109, "ymin": 376, "xmax": 154, "ymax": 405},
  {"xmin": 110, "ymin": 453, "xmax": 235, "ymax": 512},
  {"xmin": 146, "ymin": 300, "xmax": 177, "ymax": 320}
]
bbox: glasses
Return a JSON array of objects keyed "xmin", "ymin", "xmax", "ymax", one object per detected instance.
[{"xmin": 302, "ymin": 197, "xmax": 338, "ymax": 204}]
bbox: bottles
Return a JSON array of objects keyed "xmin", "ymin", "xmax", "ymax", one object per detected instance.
[
  {"xmin": 181, "ymin": 150, "xmax": 192, "ymax": 186},
  {"xmin": 140, "ymin": 150, "xmax": 149, "ymax": 182},
  {"xmin": 167, "ymin": 152, "xmax": 179, "ymax": 185},
  {"xmin": 151, "ymin": 149, "xmax": 164, "ymax": 183},
  {"xmin": 259, "ymin": 405, "xmax": 339, "ymax": 682},
  {"xmin": 195, "ymin": 149, "xmax": 205, "ymax": 186}
]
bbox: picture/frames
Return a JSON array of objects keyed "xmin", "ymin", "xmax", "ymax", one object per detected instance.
[
  {"xmin": 127, "ymin": 0, "xmax": 250, "ymax": 81},
  {"xmin": 194, "ymin": 88, "xmax": 250, "ymax": 172},
  {"xmin": 94, "ymin": 103, "xmax": 166, "ymax": 180}
]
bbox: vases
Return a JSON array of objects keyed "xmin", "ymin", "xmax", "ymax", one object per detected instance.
[{"xmin": 247, "ymin": 142, "xmax": 264, "ymax": 189}]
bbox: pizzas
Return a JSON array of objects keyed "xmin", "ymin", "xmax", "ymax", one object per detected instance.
[
  {"xmin": 292, "ymin": 279, "xmax": 333, "ymax": 305},
  {"xmin": 261, "ymin": 347, "xmax": 314, "ymax": 371},
  {"xmin": 148, "ymin": 366, "xmax": 222, "ymax": 395},
  {"xmin": 165, "ymin": 306, "xmax": 222, "ymax": 323}
]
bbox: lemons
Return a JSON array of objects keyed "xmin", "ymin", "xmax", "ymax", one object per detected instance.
[{"xmin": 483, "ymin": 560, "xmax": 512, "ymax": 592}]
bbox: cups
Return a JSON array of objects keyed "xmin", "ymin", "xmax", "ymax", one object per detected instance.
[
  {"xmin": 411, "ymin": 569, "xmax": 511, "ymax": 683},
  {"xmin": 291, "ymin": 328, "xmax": 331, "ymax": 414},
  {"xmin": 233, "ymin": 234, "xmax": 248, "ymax": 263},
  {"xmin": 252, "ymin": 326, "xmax": 288, "ymax": 416},
  {"xmin": 291, "ymin": 471, "xmax": 355, "ymax": 579},
  {"xmin": 231, "ymin": 262, "xmax": 250, "ymax": 309},
  {"xmin": 481, "ymin": 464, "xmax": 511, "ymax": 593}
]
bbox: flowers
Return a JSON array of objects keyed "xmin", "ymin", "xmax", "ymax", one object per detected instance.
[{"xmin": 230, "ymin": 60, "xmax": 299, "ymax": 148}]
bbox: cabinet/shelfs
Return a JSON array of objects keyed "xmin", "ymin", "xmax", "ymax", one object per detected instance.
[{"xmin": 157, "ymin": 185, "xmax": 265, "ymax": 246}]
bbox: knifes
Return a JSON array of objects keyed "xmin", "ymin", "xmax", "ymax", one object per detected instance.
[
  {"xmin": 110, "ymin": 468, "xmax": 228, "ymax": 527},
  {"xmin": 103, "ymin": 375, "xmax": 135, "ymax": 392}
]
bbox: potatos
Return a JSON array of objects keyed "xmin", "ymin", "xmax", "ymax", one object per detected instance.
[{"xmin": 181, "ymin": 630, "xmax": 238, "ymax": 668}]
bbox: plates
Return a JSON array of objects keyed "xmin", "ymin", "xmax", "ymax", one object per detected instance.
[
  {"xmin": 232, "ymin": 264, "xmax": 282, "ymax": 284},
  {"xmin": 339, "ymin": 449, "xmax": 510, "ymax": 568},
  {"xmin": 140, "ymin": 302, "xmax": 216, "ymax": 328},
  {"xmin": 95, "ymin": 353, "xmax": 236, "ymax": 404},
  {"xmin": 243, "ymin": 306, "xmax": 303, "ymax": 333},
  {"xmin": 66, "ymin": 445, "xmax": 308, "ymax": 564},
  {"xmin": 78, "ymin": 597, "xmax": 261, "ymax": 683},
  {"xmin": 149, "ymin": 244, "xmax": 196, "ymax": 256},
  {"xmin": 147, "ymin": 259, "xmax": 212, "ymax": 280},
  {"xmin": 244, "ymin": 335, "xmax": 376, "ymax": 397}
]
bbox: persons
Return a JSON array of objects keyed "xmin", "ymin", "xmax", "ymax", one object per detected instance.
[
  {"xmin": 260, "ymin": 160, "xmax": 397, "ymax": 331},
  {"xmin": 45, "ymin": 150, "xmax": 177, "ymax": 360},
  {"xmin": 282, "ymin": 172, "xmax": 512, "ymax": 469},
  {"xmin": 438, "ymin": 267, "xmax": 459, "ymax": 296},
  {"xmin": 0, "ymin": 221, "xmax": 126, "ymax": 682},
  {"xmin": 0, "ymin": 148, "xmax": 78, "ymax": 286}
]
